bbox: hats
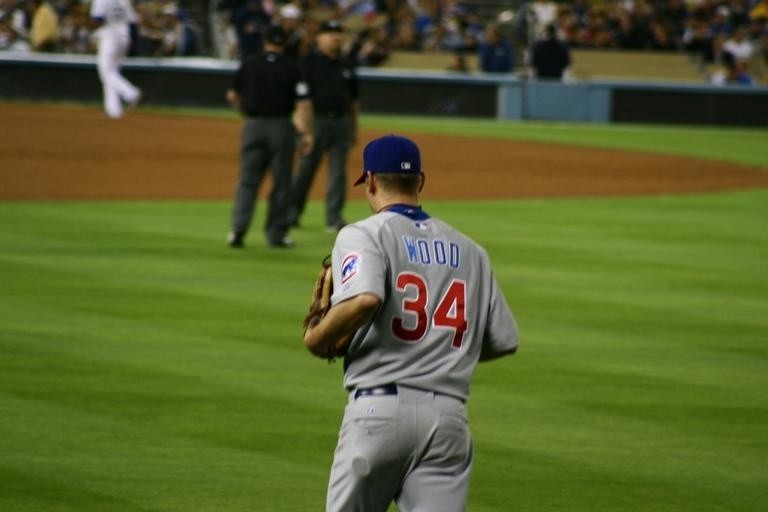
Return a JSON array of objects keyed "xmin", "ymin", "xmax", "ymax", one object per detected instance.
[
  {"xmin": 353, "ymin": 132, "xmax": 422, "ymax": 191},
  {"xmin": 265, "ymin": 25, "xmax": 286, "ymax": 45},
  {"xmin": 314, "ymin": 20, "xmax": 343, "ymax": 34}
]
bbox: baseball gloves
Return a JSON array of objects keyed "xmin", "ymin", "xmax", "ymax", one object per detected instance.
[{"xmin": 304, "ymin": 265, "xmax": 357, "ymax": 358}]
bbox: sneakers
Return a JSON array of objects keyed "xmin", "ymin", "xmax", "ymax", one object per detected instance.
[
  {"xmin": 225, "ymin": 227, "xmax": 243, "ymax": 250},
  {"xmin": 268, "ymin": 236, "xmax": 298, "ymax": 250}
]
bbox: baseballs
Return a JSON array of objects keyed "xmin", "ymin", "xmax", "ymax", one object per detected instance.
[{"xmin": 296, "ymin": 81, "xmax": 309, "ymax": 96}]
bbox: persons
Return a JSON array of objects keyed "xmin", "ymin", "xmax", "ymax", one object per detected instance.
[
  {"xmin": 301, "ymin": 134, "xmax": 518, "ymax": 512},
  {"xmin": 295, "ymin": 20, "xmax": 363, "ymax": 233},
  {"xmin": 225, "ymin": 24, "xmax": 313, "ymax": 248}
]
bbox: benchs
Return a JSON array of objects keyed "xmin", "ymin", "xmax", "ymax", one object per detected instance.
[{"xmin": 0, "ymin": 1, "xmax": 767, "ymax": 90}]
